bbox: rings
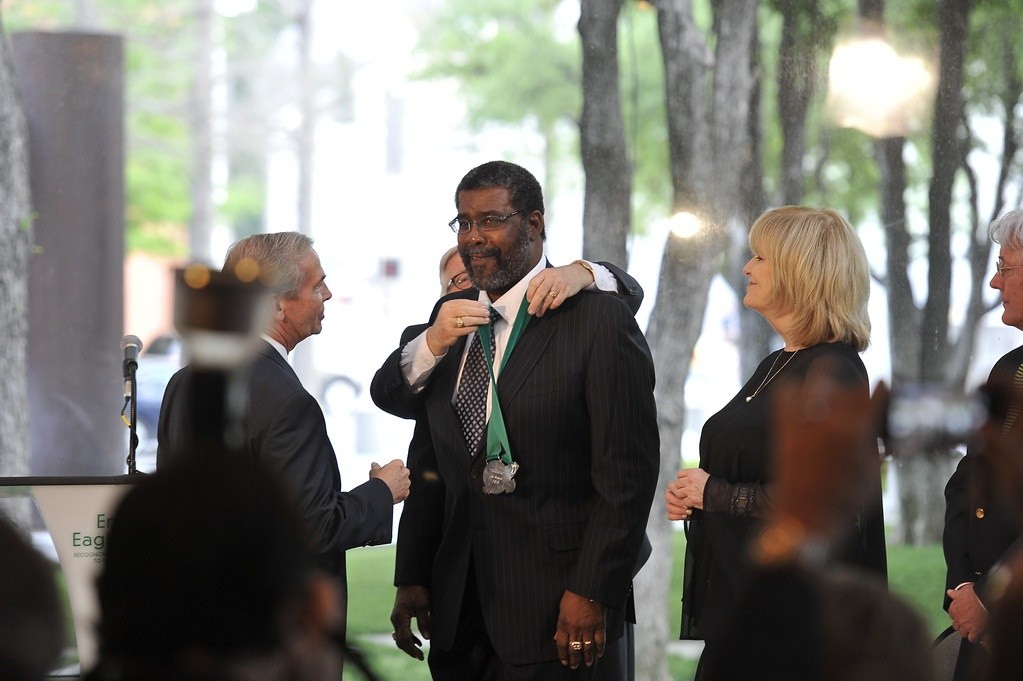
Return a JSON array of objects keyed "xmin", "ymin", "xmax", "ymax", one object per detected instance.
[
  {"xmin": 567, "ymin": 637, "xmax": 583, "ymax": 653},
  {"xmin": 456, "ymin": 316, "xmax": 464, "ymax": 329},
  {"xmin": 392, "ymin": 630, "xmax": 403, "ymax": 640},
  {"xmin": 549, "ymin": 290, "xmax": 557, "ymax": 299},
  {"xmin": 583, "ymin": 639, "xmax": 593, "ymax": 644}
]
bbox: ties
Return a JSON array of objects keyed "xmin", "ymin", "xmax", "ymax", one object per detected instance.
[
  {"xmin": 456, "ymin": 302, "xmax": 504, "ymax": 460},
  {"xmin": 999, "ymin": 363, "xmax": 1023, "ymax": 448}
]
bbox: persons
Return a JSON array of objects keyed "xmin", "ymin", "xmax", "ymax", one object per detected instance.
[
  {"xmin": 941, "ymin": 206, "xmax": 1023, "ymax": 681},
  {"xmin": 68, "ymin": 443, "xmax": 345, "ymax": 681},
  {"xmin": 666, "ymin": 203, "xmax": 893, "ymax": 678},
  {"xmin": 367, "ymin": 236, "xmax": 645, "ymax": 681},
  {"xmin": 155, "ymin": 229, "xmax": 415, "ymax": 679},
  {"xmin": 0, "ymin": 502, "xmax": 69, "ymax": 678},
  {"xmin": 684, "ymin": 554, "xmax": 937, "ymax": 678},
  {"xmin": 391, "ymin": 159, "xmax": 659, "ymax": 681}
]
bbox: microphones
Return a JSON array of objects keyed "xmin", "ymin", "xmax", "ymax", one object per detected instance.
[{"xmin": 123, "ymin": 335, "xmax": 141, "ymax": 401}]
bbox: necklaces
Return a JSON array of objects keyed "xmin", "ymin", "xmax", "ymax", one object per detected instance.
[{"xmin": 743, "ymin": 332, "xmax": 810, "ymax": 403}]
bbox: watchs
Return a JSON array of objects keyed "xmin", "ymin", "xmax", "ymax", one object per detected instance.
[{"xmin": 572, "ymin": 260, "xmax": 597, "ymax": 285}]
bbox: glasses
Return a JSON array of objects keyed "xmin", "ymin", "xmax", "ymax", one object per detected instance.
[
  {"xmin": 996, "ymin": 259, "xmax": 1023, "ymax": 277},
  {"xmin": 448, "ymin": 208, "xmax": 525, "ymax": 234},
  {"xmin": 440, "ymin": 271, "xmax": 474, "ymax": 297}
]
